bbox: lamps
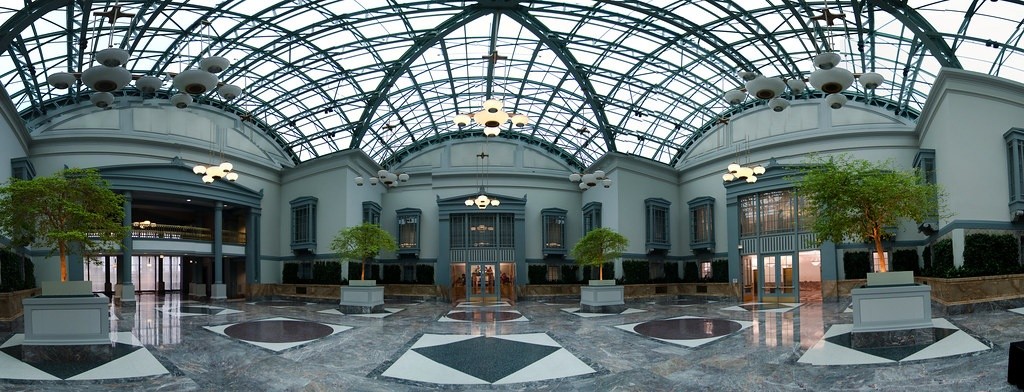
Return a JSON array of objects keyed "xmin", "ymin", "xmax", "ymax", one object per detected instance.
[
  {"xmin": 568, "ymin": 92, "xmax": 613, "ymax": 191},
  {"xmin": 470, "ymin": 225, "xmax": 494, "ymax": 233},
  {"xmin": 46, "ymin": 6, "xmax": 163, "ymax": 109},
  {"xmin": 788, "ymin": 0, "xmax": 885, "ymax": 109},
  {"xmin": 168, "ymin": 21, "xmax": 243, "ymax": 110},
  {"xmin": 546, "ymin": 241, "xmax": 561, "ymax": 247},
  {"xmin": 465, "ymin": 139, "xmax": 500, "ymax": 209},
  {"xmin": 452, "ymin": 14, "xmax": 529, "ymax": 137},
  {"xmin": 725, "ymin": 67, "xmax": 789, "ymax": 112},
  {"xmin": 401, "ymin": 219, "xmax": 416, "ymax": 248},
  {"xmin": 355, "ymin": 81, "xmax": 409, "ymax": 189},
  {"xmin": 132, "ymin": 217, "xmax": 156, "ymax": 229},
  {"xmin": 193, "ymin": 119, "xmax": 238, "ymax": 185},
  {"xmin": 722, "ymin": 132, "xmax": 766, "ymax": 185},
  {"xmin": 473, "ymin": 236, "xmax": 489, "ymax": 246}
]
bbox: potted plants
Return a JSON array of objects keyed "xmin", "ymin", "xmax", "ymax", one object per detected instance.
[
  {"xmin": 328, "ymin": 223, "xmax": 400, "ymax": 308},
  {"xmin": 778, "ymin": 150, "xmax": 961, "ymax": 334},
  {"xmin": 566, "ymin": 227, "xmax": 632, "ymax": 307},
  {"xmin": 0, "ymin": 164, "xmax": 134, "ymax": 344}
]
